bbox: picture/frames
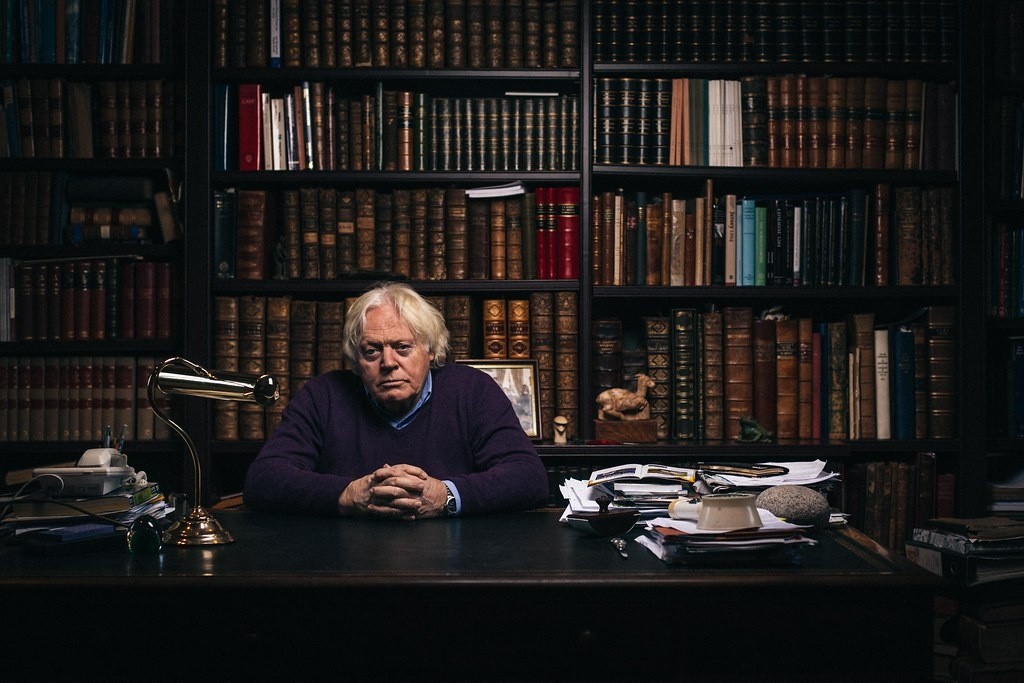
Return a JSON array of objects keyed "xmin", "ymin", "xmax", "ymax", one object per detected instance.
[{"xmin": 453, "ymin": 358, "xmax": 543, "ymax": 441}]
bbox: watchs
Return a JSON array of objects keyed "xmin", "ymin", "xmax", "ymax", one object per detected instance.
[{"xmin": 445, "ymin": 484, "xmax": 457, "ymax": 517}]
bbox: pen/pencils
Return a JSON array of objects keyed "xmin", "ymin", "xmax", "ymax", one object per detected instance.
[
  {"xmin": 104, "ymin": 425, "xmax": 111, "ymax": 448},
  {"xmin": 113, "ymin": 424, "xmax": 127, "ymax": 454}
]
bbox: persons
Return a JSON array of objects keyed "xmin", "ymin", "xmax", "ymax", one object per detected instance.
[{"xmin": 242, "ymin": 280, "xmax": 549, "ymax": 522}]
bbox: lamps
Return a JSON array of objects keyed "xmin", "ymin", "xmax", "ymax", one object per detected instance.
[{"xmin": 145, "ymin": 356, "xmax": 281, "ymax": 547}]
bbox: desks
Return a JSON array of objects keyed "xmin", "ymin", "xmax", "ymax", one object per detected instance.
[{"xmin": 0, "ymin": 507, "xmax": 939, "ymax": 683}]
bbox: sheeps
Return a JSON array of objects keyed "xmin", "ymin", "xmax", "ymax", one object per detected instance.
[{"xmin": 596, "ymin": 373, "xmax": 656, "ymax": 421}]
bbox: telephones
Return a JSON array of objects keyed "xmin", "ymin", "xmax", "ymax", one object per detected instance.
[{"xmin": 33, "ymin": 448, "xmax": 136, "ymax": 497}]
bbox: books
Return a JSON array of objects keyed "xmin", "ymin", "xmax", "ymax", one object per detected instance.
[
  {"xmin": 853, "ymin": 451, "xmax": 1024, "ymax": 591},
  {"xmin": 0, "ymin": 461, "xmax": 178, "ymax": 544},
  {"xmin": 0, "ymin": 0, "xmax": 1024, "ymax": 449},
  {"xmin": 559, "ymin": 458, "xmax": 852, "ymax": 564},
  {"xmin": 208, "ymin": 479, "xmax": 244, "ymax": 512}
]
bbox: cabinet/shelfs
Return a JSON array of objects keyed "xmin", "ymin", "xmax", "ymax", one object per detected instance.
[{"xmin": 0, "ymin": 0, "xmax": 1024, "ymax": 528}]
